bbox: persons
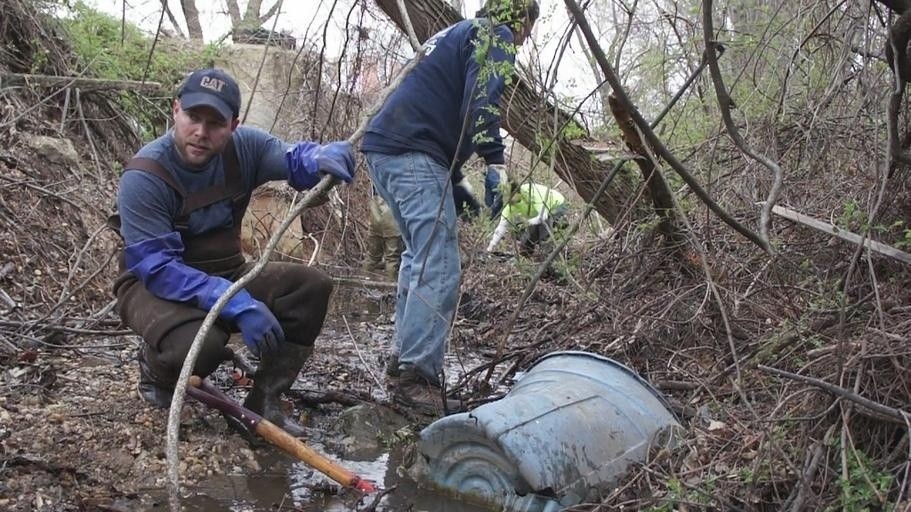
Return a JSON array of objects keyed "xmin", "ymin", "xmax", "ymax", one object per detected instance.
[
  {"xmin": 359, "ymin": 1, "xmax": 542, "ymax": 403},
  {"xmin": 486, "ymin": 179, "xmax": 568, "ymax": 259},
  {"xmin": 362, "ymin": 186, "xmax": 403, "ymax": 275},
  {"xmin": 109, "ymin": 67, "xmax": 358, "ymax": 441}
]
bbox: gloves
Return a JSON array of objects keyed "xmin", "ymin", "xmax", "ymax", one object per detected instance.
[
  {"xmin": 284, "ymin": 140, "xmax": 357, "ymax": 192},
  {"xmin": 453, "ymin": 175, "xmax": 483, "ymax": 226},
  {"xmin": 484, "ymin": 163, "xmax": 508, "ymax": 222},
  {"xmin": 122, "ymin": 231, "xmax": 286, "ymax": 360}
]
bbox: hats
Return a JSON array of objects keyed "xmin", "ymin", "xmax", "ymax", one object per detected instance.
[{"xmin": 177, "ymin": 67, "xmax": 241, "ymax": 121}]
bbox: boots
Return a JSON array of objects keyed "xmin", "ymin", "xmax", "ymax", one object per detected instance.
[
  {"xmin": 135, "ymin": 342, "xmax": 181, "ymax": 408},
  {"xmin": 240, "ymin": 339, "xmax": 314, "ymax": 441}
]
